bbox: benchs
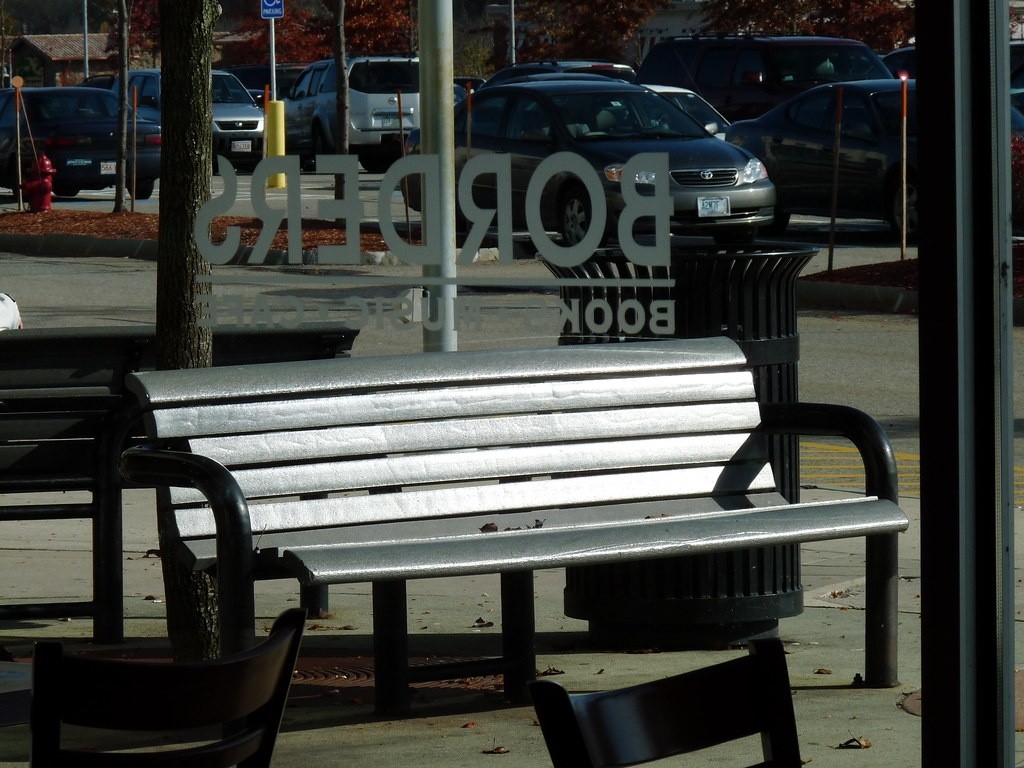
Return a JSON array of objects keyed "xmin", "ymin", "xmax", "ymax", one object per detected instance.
[
  {"xmin": 110, "ymin": 314, "xmax": 908, "ymax": 691},
  {"xmin": 0, "ymin": 321, "xmax": 359, "ymax": 642}
]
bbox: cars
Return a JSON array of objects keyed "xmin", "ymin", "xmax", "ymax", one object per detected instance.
[
  {"xmin": 395, "ymin": 78, "xmax": 776, "ymax": 257},
  {"xmin": 0, "ymin": 86, "xmax": 163, "ymax": 198},
  {"xmin": 882, "ymin": 38, "xmax": 1024, "ymax": 91},
  {"xmin": 610, "ymin": 83, "xmax": 732, "ymax": 141},
  {"xmin": 452, "ymin": 77, "xmax": 486, "ymax": 91},
  {"xmin": 475, "ymin": 58, "xmax": 638, "ymax": 88},
  {"xmin": 724, "ymin": 78, "xmax": 1024, "ymax": 238}
]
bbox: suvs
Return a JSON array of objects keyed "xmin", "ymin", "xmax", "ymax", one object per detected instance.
[
  {"xmin": 111, "ymin": 68, "xmax": 267, "ymax": 172},
  {"xmin": 637, "ymin": 31, "xmax": 894, "ymax": 125},
  {"xmin": 73, "ymin": 72, "xmax": 117, "ymax": 88},
  {"xmin": 280, "ymin": 56, "xmax": 422, "ymax": 172}
]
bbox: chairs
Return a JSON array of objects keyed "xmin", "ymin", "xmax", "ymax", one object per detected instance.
[
  {"xmin": 29, "ymin": 608, "xmax": 308, "ymax": 768},
  {"xmin": 586, "ymin": 107, "xmax": 635, "ymax": 136},
  {"xmin": 528, "ymin": 637, "xmax": 804, "ymax": 768}
]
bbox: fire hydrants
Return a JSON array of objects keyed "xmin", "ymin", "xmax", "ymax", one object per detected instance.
[{"xmin": 26, "ymin": 153, "xmax": 58, "ymax": 213}]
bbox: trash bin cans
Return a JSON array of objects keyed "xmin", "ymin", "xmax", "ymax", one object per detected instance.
[{"xmin": 530, "ymin": 226, "xmax": 823, "ymax": 651}]
